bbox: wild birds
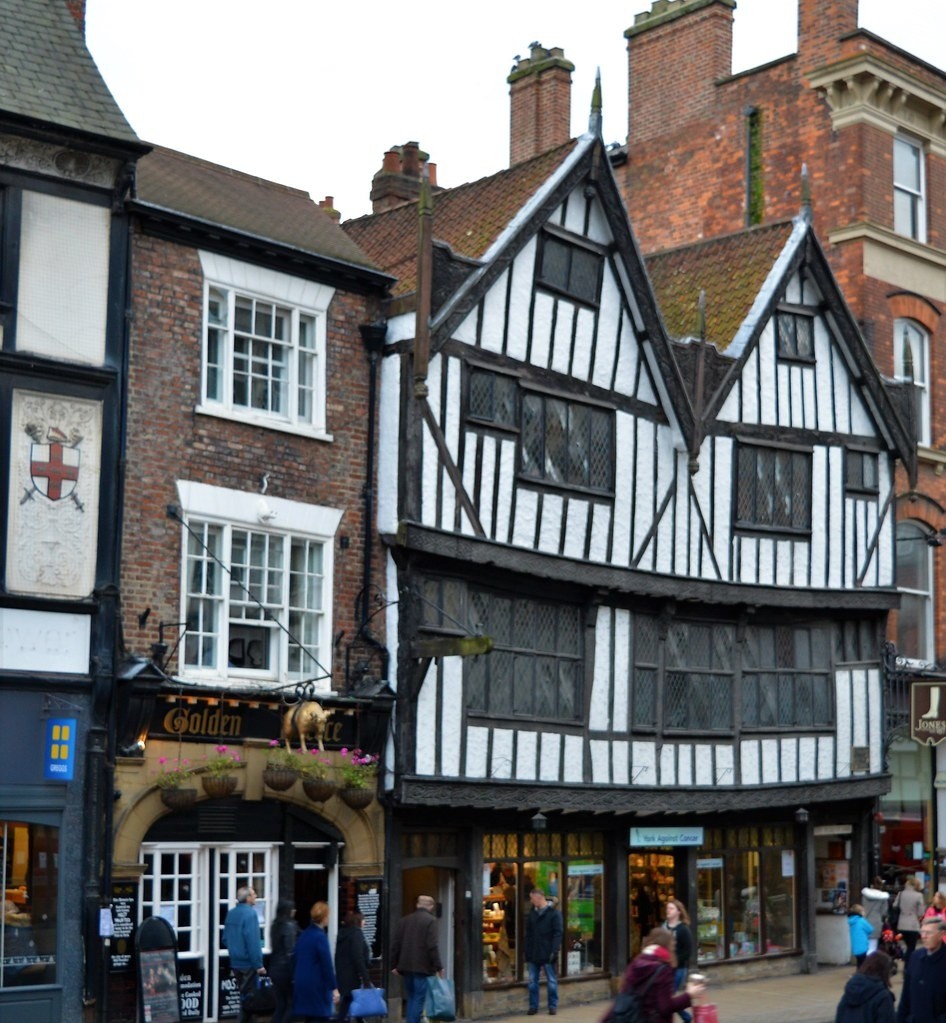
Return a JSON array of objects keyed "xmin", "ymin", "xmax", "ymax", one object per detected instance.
[{"xmin": 120, "ymin": 741, "xmax": 146, "ymax": 758}]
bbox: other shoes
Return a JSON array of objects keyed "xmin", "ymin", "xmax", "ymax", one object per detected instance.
[
  {"xmin": 528, "ymin": 1008, "xmax": 538, "ymax": 1015},
  {"xmin": 549, "ymin": 1007, "xmax": 556, "ymax": 1014}
]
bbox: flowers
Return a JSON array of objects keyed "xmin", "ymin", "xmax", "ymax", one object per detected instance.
[
  {"xmin": 333, "ymin": 748, "xmax": 380, "ymax": 790},
  {"xmin": 192, "ymin": 745, "xmax": 241, "ymax": 776},
  {"xmin": 150, "ymin": 757, "xmax": 192, "ymax": 790},
  {"xmin": 295, "ymin": 748, "xmax": 338, "ymax": 785},
  {"xmin": 255, "ymin": 740, "xmax": 301, "ymax": 770}
]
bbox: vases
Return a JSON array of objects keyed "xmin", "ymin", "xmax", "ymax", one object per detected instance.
[
  {"xmin": 200, "ymin": 776, "xmax": 238, "ymax": 798},
  {"xmin": 301, "ymin": 781, "xmax": 335, "ymax": 804},
  {"xmin": 160, "ymin": 789, "xmax": 197, "ymax": 809},
  {"xmin": 336, "ymin": 789, "xmax": 374, "ymax": 811},
  {"xmin": 261, "ymin": 770, "xmax": 298, "ymax": 791}
]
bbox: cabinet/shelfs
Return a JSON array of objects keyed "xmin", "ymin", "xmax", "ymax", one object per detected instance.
[
  {"xmin": 482, "ymin": 895, "xmax": 508, "ymax": 950},
  {"xmin": 629, "ymin": 864, "xmax": 674, "ymax": 918}
]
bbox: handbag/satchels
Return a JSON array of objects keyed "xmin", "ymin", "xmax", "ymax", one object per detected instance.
[
  {"xmin": 611, "ymin": 962, "xmax": 669, "ymax": 1023},
  {"xmin": 426, "ymin": 971, "xmax": 456, "ymax": 1021},
  {"xmin": 890, "ymin": 906, "xmax": 899, "ymax": 926},
  {"xmin": 349, "ymin": 981, "xmax": 388, "ymax": 1017},
  {"xmin": 239, "ymin": 972, "xmax": 276, "ymax": 1016}
]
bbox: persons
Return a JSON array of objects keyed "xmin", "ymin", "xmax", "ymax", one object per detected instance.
[
  {"xmin": 222, "ymin": 886, "xmax": 266, "ymax": 1023},
  {"xmin": 390, "ymin": 895, "xmax": 444, "ymax": 1023},
  {"xmin": 269, "ymin": 900, "xmax": 301, "ymax": 1023},
  {"xmin": 524, "ymin": 889, "xmax": 563, "ymax": 1015},
  {"xmin": 836, "ymin": 874, "xmax": 946, "ymax": 1023},
  {"xmin": 617, "ymin": 927, "xmax": 706, "ymax": 1023},
  {"xmin": 503, "ymin": 886, "xmax": 516, "ymax": 947},
  {"xmin": 661, "ymin": 899, "xmax": 694, "ymax": 1023},
  {"xmin": 290, "ymin": 902, "xmax": 340, "ymax": 1023},
  {"xmin": 333, "ymin": 909, "xmax": 372, "ymax": 1023}
]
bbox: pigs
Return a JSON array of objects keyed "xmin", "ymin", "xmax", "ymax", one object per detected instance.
[{"xmin": 281, "ymin": 700, "xmax": 335, "ymax": 752}]
